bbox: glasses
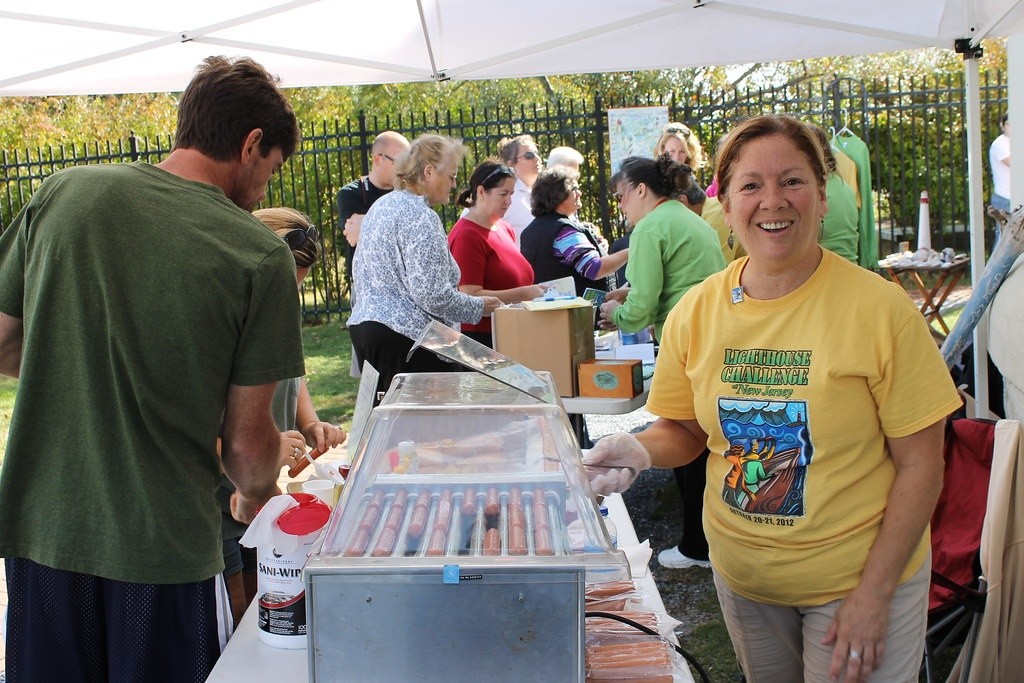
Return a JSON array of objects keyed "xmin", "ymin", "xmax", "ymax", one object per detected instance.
[
  {"xmin": 515, "ymin": 151, "xmax": 537, "ymax": 160},
  {"xmin": 658, "ymin": 128, "xmax": 690, "ymax": 142},
  {"xmin": 430, "ymin": 161, "xmax": 457, "ymax": 184},
  {"xmin": 612, "ymin": 179, "xmax": 645, "ymax": 202},
  {"xmin": 280, "ymin": 225, "xmax": 319, "ymax": 250},
  {"xmin": 570, "ymin": 184, "xmax": 584, "ymax": 194},
  {"xmin": 481, "ymin": 165, "xmax": 515, "ymax": 184}
]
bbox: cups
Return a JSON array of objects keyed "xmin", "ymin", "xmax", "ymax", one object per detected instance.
[
  {"xmin": 339, "ymin": 465, "xmax": 350, "ymax": 478},
  {"xmin": 305, "ymin": 451, "xmax": 345, "ymax": 505},
  {"xmin": 302, "ymin": 480, "xmax": 334, "ymax": 506}
]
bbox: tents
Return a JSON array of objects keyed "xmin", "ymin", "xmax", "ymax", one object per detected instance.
[{"xmin": 1, "ymin": 1, "xmax": 1023, "ymax": 417}]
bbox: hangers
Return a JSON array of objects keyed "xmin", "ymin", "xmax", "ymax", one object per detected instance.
[
  {"xmin": 834, "ymin": 107, "xmax": 855, "ymax": 138},
  {"xmin": 825, "ymin": 127, "xmax": 840, "ymax": 154}
]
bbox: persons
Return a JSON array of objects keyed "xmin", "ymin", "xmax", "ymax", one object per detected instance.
[
  {"xmin": 598, "ymin": 154, "xmax": 728, "ymax": 569},
  {"xmin": 0, "ymin": 56, "xmax": 305, "ymax": 683},
  {"xmin": 654, "ymin": 122, "xmax": 702, "ymax": 171},
  {"xmin": 216, "ymin": 207, "xmax": 346, "ymax": 628},
  {"xmin": 346, "ymin": 135, "xmax": 504, "ymax": 405},
  {"xmin": 445, "ymin": 162, "xmax": 534, "ymax": 348},
  {"xmin": 499, "ymin": 136, "xmax": 631, "ymax": 328},
  {"xmin": 337, "ymin": 131, "xmax": 410, "ymax": 278},
  {"xmin": 580, "ymin": 115, "xmax": 963, "ymax": 683},
  {"xmin": 989, "ymin": 117, "xmax": 1011, "ymax": 240}
]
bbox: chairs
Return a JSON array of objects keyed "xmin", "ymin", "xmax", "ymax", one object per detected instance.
[{"xmin": 920, "ymin": 390, "xmax": 1020, "ymax": 683}]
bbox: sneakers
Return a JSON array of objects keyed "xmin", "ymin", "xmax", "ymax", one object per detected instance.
[{"xmin": 658, "ymin": 546, "xmax": 711, "ymax": 569}]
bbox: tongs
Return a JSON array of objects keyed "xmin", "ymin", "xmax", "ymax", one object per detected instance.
[{"xmin": 534, "ymin": 457, "xmax": 638, "ymax": 483}]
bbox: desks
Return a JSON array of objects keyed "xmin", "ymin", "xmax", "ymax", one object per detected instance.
[
  {"xmin": 559, "ymin": 375, "xmax": 654, "ymax": 449},
  {"xmin": 870, "ymin": 257, "xmax": 970, "ymax": 346},
  {"xmin": 206, "ymin": 395, "xmax": 699, "ymax": 683}
]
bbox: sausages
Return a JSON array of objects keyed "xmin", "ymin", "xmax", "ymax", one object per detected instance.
[
  {"xmin": 288, "ymin": 444, "xmax": 329, "ymax": 478},
  {"xmin": 585, "ymin": 578, "xmax": 674, "ymax": 683},
  {"xmin": 343, "ymin": 486, "xmax": 556, "ymax": 557}
]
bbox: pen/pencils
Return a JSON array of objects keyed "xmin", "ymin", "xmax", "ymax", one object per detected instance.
[{"xmin": 541, "ymin": 296, "xmax": 575, "ymax": 302}]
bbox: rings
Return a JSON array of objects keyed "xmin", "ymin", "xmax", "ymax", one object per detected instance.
[
  {"xmin": 849, "ymin": 651, "xmax": 862, "ymax": 657},
  {"xmin": 292, "ymin": 447, "xmax": 299, "ymax": 458}
]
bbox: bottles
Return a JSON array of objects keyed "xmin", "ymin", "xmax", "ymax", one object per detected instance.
[{"xmin": 585, "ymin": 507, "xmax": 617, "ymax": 579}]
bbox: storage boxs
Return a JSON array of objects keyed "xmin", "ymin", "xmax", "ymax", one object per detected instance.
[
  {"xmin": 577, "ymin": 358, "xmax": 643, "ymax": 398},
  {"xmin": 491, "ymin": 304, "xmax": 593, "ymax": 396}
]
